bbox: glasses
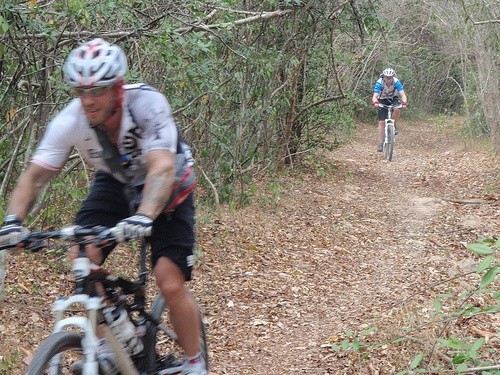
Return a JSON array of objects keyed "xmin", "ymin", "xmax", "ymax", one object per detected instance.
[{"xmin": 71, "ymin": 86, "xmax": 111, "ymax": 98}]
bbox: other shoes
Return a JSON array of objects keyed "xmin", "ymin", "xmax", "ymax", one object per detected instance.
[
  {"xmin": 377, "ymin": 144, "xmax": 383, "ymax": 151},
  {"xmin": 71, "ymin": 344, "xmax": 118, "ymax": 375},
  {"xmin": 182, "ymin": 356, "xmax": 206, "ymax": 375},
  {"xmin": 395, "ymin": 128, "xmax": 398, "ymax": 135}
]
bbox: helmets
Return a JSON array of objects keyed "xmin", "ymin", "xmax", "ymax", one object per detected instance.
[
  {"xmin": 64, "ymin": 37, "xmax": 127, "ymax": 87},
  {"xmin": 383, "ymin": 68, "xmax": 395, "ymax": 77}
]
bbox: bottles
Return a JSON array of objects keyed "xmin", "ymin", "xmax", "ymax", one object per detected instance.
[{"xmin": 111, "ymin": 308, "xmax": 143, "ymax": 356}]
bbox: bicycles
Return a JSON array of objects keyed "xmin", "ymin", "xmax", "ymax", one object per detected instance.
[
  {"xmin": 0, "ymin": 221, "xmax": 210, "ymax": 375},
  {"xmin": 370, "ymin": 102, "xmax": 411, "ymax": 161}
]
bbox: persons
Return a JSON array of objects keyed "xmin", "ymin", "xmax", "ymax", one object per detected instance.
[
  {"xmin": 372, "ymin": 67, "xmax": 409, "ymax": 152},
  {"xmin": 0, "ymin": 38, "xmax": 208, "ymax": 375}
]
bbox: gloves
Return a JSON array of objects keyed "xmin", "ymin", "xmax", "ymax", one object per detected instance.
[
  {"xmin": 0, "ymin": 215, "xmax": 29, "ymax": 247},
  {"xmin": 116, "ymin": 215, "xmax": 152, "ymax": 243}
]
